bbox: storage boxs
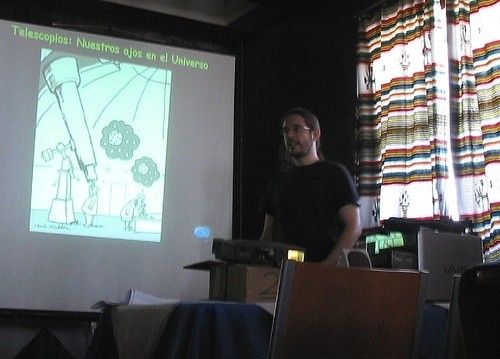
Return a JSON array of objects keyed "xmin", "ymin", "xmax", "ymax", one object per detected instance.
[{"xmin": 183, "ymin": 258, "xmax": 282, "ymax": 302}]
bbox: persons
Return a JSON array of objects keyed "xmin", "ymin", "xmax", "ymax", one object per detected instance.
[{"xmin": 260, "ymin": 105, "xmax": 362, "ymax": 265}]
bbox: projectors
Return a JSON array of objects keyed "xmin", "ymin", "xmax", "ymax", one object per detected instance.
[{"xmin": 211, "ymin": 238, "xmax": 306, "ymax": 267}]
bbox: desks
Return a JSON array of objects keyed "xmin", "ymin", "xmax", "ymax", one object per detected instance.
[{"xmin": 88, "ymin": 301, "xmax": 276, "ymax": 359}]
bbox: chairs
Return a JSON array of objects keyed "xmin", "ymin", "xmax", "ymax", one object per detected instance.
[
  {"xmin": 265, "ymin": 259, "xmax": 421, "ymax": 359},
  {"xmin": 443, "ymin": 260, "xmax": 500, "ymax": 359}
]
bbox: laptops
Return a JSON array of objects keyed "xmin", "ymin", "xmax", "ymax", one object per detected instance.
[{"xmin": 418, "ymin": 230, "xmax": 483, "ymax": 302}]
